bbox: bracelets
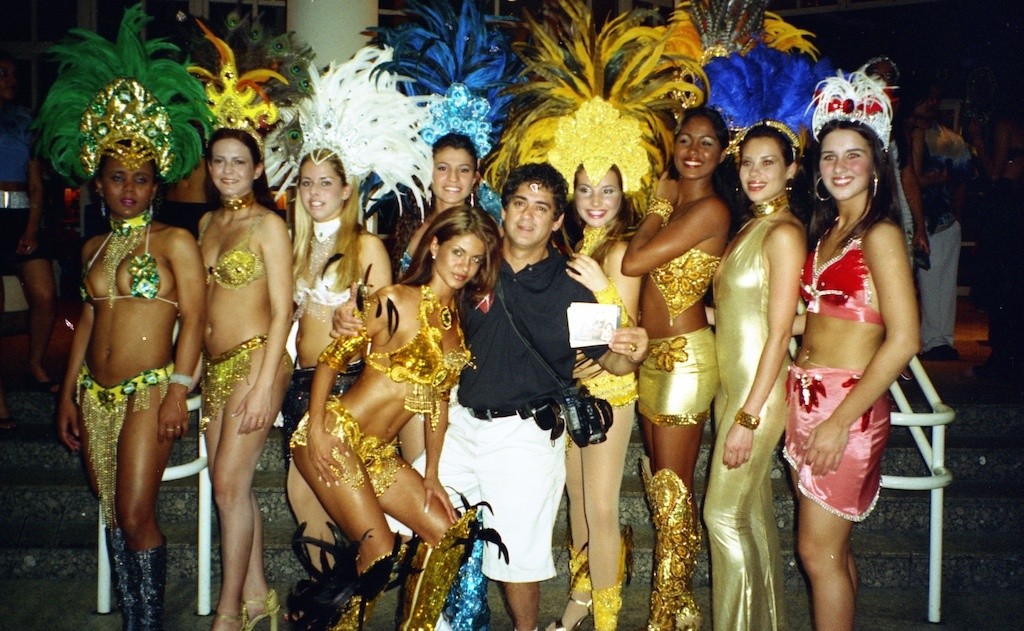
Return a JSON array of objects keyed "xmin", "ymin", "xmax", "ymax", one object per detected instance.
[
  {"xmin": 646, "ymin": 198, "xmax": 674, "ymax": 226},
  {"xmin": 735, "ymin": 408, "xmax": 762, "ymax": 432}
]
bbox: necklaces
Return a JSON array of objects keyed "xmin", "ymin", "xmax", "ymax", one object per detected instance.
[
  {"xmin": 426, "ymin": 286, "xmax": 457, "ymax": 332},
  {"xmin": 751, "ymin": 195, "xmax": 792, "ymax": 219},
  {"xmin": 308, "ymin": 218, "xmax": 345, "ymax": 275},
  {"xmin": 105, "ymin": 209, "xmax": 154, "ymax": 310},
  {"xmin": 218, "ymin": 193, "xmax": 257, "ymax": 213}
]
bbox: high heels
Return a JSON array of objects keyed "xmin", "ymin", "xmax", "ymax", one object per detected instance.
[
  {"xmin": 242, "ymin": 587, "xmax": 281, "ymax": 631},
  {"xmin": 22, "ymin": 360, "xmax": 61, "ymax": 394},
  {"xmin": 0, "ymin": 416, "xmax": 18, "ymax": 434},
  {"xmin": 544, "ymin": 596, "xmax": 594, "ymax": 631},
  {"xmin": 215, "ymin": 600, "xmax": 248, "ymax": 631}
]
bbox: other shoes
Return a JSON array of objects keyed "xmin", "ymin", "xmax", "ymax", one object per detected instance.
[{"xmin": 921, "ymin": 345, "xmax": 959, "ymax": 361}]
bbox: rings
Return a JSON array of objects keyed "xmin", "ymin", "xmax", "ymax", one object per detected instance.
[{"xmin": 630, "ymin": 343, "xmax": 638, "ymax": 354}]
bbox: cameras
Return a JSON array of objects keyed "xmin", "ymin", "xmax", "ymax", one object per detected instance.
[{"xmin": 554, "ymin": 384, "xmax": 610, "ymax": 449}]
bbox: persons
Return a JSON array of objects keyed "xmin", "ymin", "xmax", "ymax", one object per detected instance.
[
  {"xmin": 279, "ymin": 207, "xmax": 501, "ymax": 631},
  {"xmin": 58, "ymin": 139, "xmax": 209, "ymax": 631},
  {"xmin": 328, "ymin": 161, "xmax": 651, "ymax": 631},
  {"xmin": 866, "ymin": 39, "xmax": 1024, "ymax": 389},
  {"xmin": 700, "ymin": 118, "xmax": 810, "ymax": 631},
  {"xmin": 543, "ymin": 160, "xmax": 646, "ymax": 631},
  {"xmin": 786, "ymin": 71, "xmax": 924, "ymax": 631},
  {"xmin": 621, "ymin": 104, "xmax": 732, "ymax": 631},
  {"xmin": 0, "ymin": 33, "xmax": 226, "ymax": 435},
  {"xmin": 396, "ymin": 135, "xmax": 506, "ymax": 284},
  {"xmin": 287, "ymin": 150, "xmax": 392, "ymax": 583},
  {"xmin": 192, "ymin": 129, "xmax": 296, "ymax": 631}
]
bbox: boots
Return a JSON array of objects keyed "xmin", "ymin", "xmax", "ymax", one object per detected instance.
[
  {"xmin": 637, "ymin": 454, "xmax": 704, "ymax": 631},
  {"xmin": 130, "ymin": 537, "xmax": 167, "ymax": 631},
  {"xmin": 325, "ymin": 543, "xmax": 408, "ymax": 631},
  {"xmin": 644, "ymin": 468, "xmax": 696, "ymax": 631},
  {"xmin": 103, "ymin": 527, "xmax": 143, "ymax": 631},
  {"xmin": 397, "ymin": 507, "xmax": 478, "ymax": 631}
]
